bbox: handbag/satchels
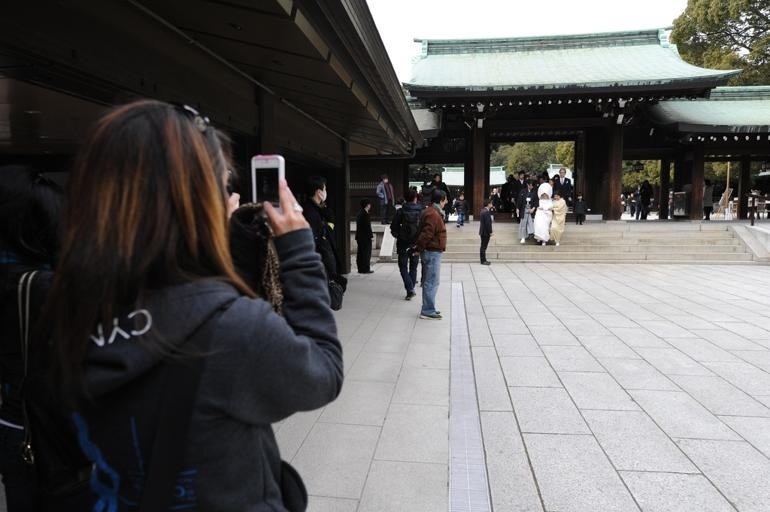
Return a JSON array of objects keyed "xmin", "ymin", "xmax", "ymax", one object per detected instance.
[{"xmin": 0, "ymin": 432, "xmax": 39, "ymax": 512}]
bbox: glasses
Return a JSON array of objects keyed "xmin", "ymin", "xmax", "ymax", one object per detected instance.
[{"xmin": 179, "ymin": 103, "xmax": 210, "ymax": 139}]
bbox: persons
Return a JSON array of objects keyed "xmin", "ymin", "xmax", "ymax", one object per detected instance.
[
  {"xmin": 390, "ymin": 190, "xmax": 446, "ymax": 319},
  {"xmin": 703, "ymin": 178, "xmax": 714, "ymax": 220},
  {"xmin": 355, "ymin": 198, "xmax": 374, "ymax": 273},
  {"xmin": 493, "ymin": 166, "xmax": 586, "ymax": 247},
  {"xmin": 376, "ymin": 174, "xmax": 468, "ymax": 226},
  {"xmin": 479, "ymin": 199, "xmax": 494, "ymax": 265},
  {"xmin": 620, "ymin": 180, "xmax": 654, "ymax": 220},
  {"xmin": 1, "ymin": 101, "xmax": 343, "ymax": 511}
]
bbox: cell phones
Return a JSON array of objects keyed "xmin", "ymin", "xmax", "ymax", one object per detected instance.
[{"xmin": 250, "ymin": 154, "xmax": 285, "ymax": 223}]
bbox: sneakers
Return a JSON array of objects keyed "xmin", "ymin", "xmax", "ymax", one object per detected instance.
[
  {"xmin": 556, "ymin": 242, "xmax": 559, "ymax": 246},
  {"xmin": 405, "ymin": 293, "xmax": 416, "ymax": 299},
  {"xmin": 542, "ymin": 242, "xmax": 546, "ymax": 246},
  {"xmin": 420, "ymin": 311, "xmax": 441, "ymax": 319},
  {"xmin": 520, "ymin": 238, "xmax": 526, "ymax": 244},
  {"xmin": 482, "ymin": 262, "xmax": 491, "ymax": 265}
]
